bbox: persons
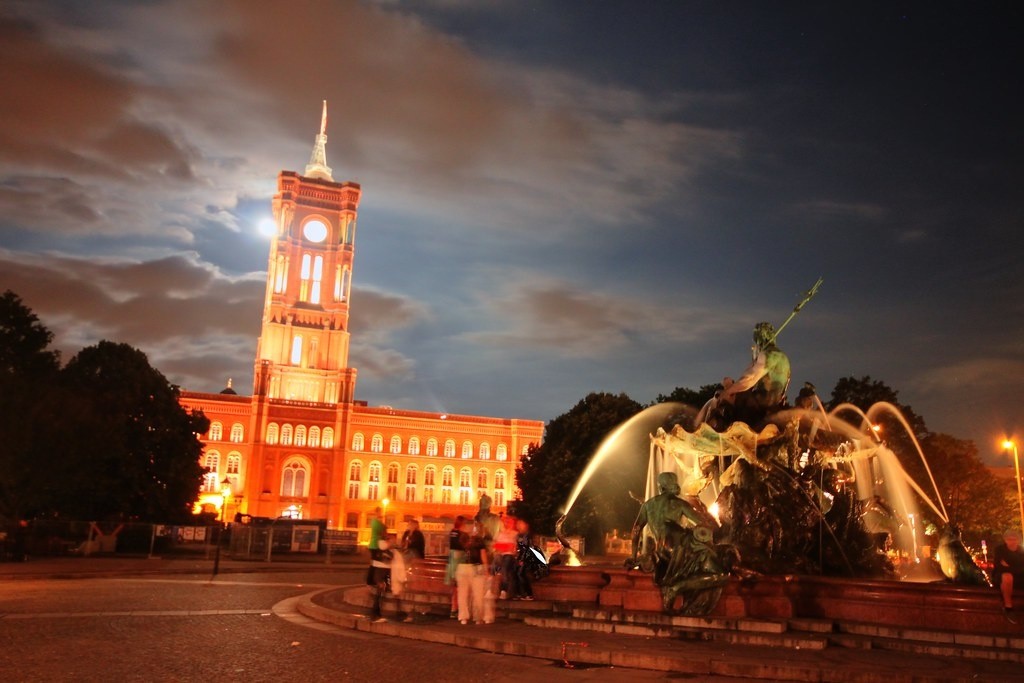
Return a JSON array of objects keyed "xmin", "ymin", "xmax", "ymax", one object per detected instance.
[
  {"xmin": 366, "ymin": 515, "xmax": 556, "ymax": 624},
  {"xmin": 996, "ymin": 529, "xmax": 1024, "ymax": 627},
  {"xmin": 631, "ymin": 472, "xmax": 706, "ymax": 570},
  {"xmin": 705, "ymin": 322, "xmax": 791, "ymax": 432}
]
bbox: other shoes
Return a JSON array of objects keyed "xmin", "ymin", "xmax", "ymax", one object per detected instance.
[
  {"xmin": 403, "ymin": 615, "xmax": 414, "ymax": 622},
  {"xmin": 371, "ymin": 618, "xmax": 387, "ymax": 624},
  {"xmin": 461, "ymin": 620, "xmax": 468, "ymax": 625},
  {"xmin": 513, "ymin": 597, "xmax": 520, "ymax": 601},
  {"xmin": 486, "ymin": 621, "xmax": 495, "ymax": 624},
  {"xmin": 450, "ymin": 612, "xmax": 456, "ymax": 619},
  {"xmin": 520, "ymin": 596, "xmax": 533, "ymax": 601},
  {"xmin": 476, "ymin": 621, "xmax": 483, "ymax": 624},
  {"xmin": 499, "ymin": 591, "xmax": 507, "ymax": 598}
]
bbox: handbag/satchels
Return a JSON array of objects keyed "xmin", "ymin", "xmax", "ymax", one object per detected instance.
[
  {"xmin": 526, "ymin": 546, "xmax": 550, "ymax": 579},
  {"xmin": 452, "ymin": 586, "xmax": 458, "ymax": 612},
  {"xmin": 484, "ymin": 590, "xmax": 494, "ymax": 621}
]
